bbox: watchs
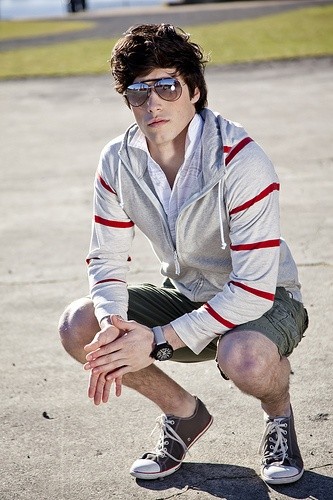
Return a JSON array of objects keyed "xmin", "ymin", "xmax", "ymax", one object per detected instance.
[{"xmin": 150, "ymin": 325, "xmax": 174, "ymax": 362}]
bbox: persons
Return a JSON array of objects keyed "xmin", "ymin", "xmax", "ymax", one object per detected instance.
[{"xmin": 57, "ymin": 22, "xmax": 310, "ymax": 485}]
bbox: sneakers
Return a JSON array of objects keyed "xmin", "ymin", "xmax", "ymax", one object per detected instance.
[
  {"xmin": 256, "ymin": 403, "xmax": 305, "ymax": 485},
  {"xmin": 128, "ymin": 395, "xmax": 214, "ymax": 480}
]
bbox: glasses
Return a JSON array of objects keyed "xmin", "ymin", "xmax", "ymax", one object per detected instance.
[{"xmin": 120, "ymin": 76, "xmax": 186, "ymax": 107}]
